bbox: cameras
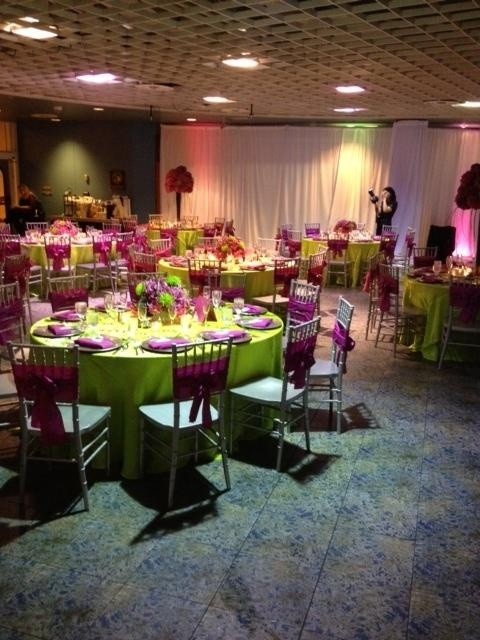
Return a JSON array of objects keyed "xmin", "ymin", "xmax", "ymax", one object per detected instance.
[{"xmin": 368, "ymin": 189, "xmax": 378, "ymax": 203}]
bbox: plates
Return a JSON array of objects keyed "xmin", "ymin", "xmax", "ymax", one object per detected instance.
[
  {"xmin": 163, "ymin": 253, "xmax": 292, "ymax": 271},
  {"xmin": 33, "ymin": 297, "xmax": 282, "ymax": 353},
  {"xmin": 406, "ymin": 265, "xmax": 444, "ymax": 284}
]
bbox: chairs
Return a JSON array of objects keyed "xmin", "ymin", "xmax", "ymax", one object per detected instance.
[
  {"xmin": 279, "ymin": 281, "xmax": 321, "ymax": 364},
  {"xmin": 365, "ymin": 247, "xmax": 480, "ymax": 372},
  {"xmin": 137, "ymin": 335, "xmax": 234, "ymax": 505},
  {"xmin": 47, "ymin": 273, "xmax": 90, "ymax": 312},
  {"xmin": 125, "ymin": 272, "xmax": 169, "ymax": 292},
  {"xmin": 1, "ymin": 282, "xmax": 36, "ymax": 364},
  {"xmin": 2, "ymin": 215, "xmax": 401, "ymax": 305},
  {"xmin": 228, "ymin": 315, "xmax": 320, "ymax": 470},
  {"xmin": 276, "ymin": 295, "xmax": 353, "ymax": 436},
  {"xmin": 7, "ymin": 340, "xmax": 112, "ymax": 508},
  {"xmin": 8, "ymin": 257, "xmax": 33, "ymax": 333}
]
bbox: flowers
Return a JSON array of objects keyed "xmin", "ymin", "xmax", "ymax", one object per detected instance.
[{"xmin": 138, "ymin": 275, "xmax": 186, "ymax": 319}]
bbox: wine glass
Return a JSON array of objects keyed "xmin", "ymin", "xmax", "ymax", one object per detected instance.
[
  {"xmin": 73, "ymin": 285, "xmax": 245, "ymax": 334},
  {"xmin": 434, "ymin": 252, "xmax": 462, "ymax": 277},
  {"xmin": 25, "ymin": 225, "xmax": 103, "ymax": 244},
  {"xmin": 184, "ymin": 245, "xmax": 268, "ymax": 263},
  {"xmin": 321, "ymin": 227, "xmax": 372, "ymax": 240}
]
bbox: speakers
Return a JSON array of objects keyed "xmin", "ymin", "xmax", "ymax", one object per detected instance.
[{"xmin": 426, "ymin": 225, "xmax": 455, "ymax": 260}]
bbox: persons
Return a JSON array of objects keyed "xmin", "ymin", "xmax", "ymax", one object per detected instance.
[
  {"xmin": 14, "ymin": 183, "xmax": 46, "ymax": 222},
  {"xmin": 375, "ymin": 186, "xmax": 398, "ymax": 236}
]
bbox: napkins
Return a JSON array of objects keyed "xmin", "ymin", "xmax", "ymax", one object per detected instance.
[
  {"xmin": 147, "ymin": 335, "xmax": 189, "ymax": 349},
  {"xmin": 75, "ymin": 335, "xmax": 113, "ymax": 349},
  {"xmin": 252, "ymin": 318, "xmax": 272, "ymax": 327},
  {"xmin": 96, "ymin": 301, "xmax": 121, "ymax": 310},
  {"xmin": 214, "ymin": 330, "xmax": 245, "ymax": 342},
  {"xmin": 187, "ymin": 298, "xmax": 224, "ymax": 304},
  {"xmin": 243, "ymin": 304, "xmax": 263, "ymax": 314},
  {"xmin": 54, "ymin": 310, "xmax": 79, "ymax": 321},
  {"xmin": 47, "ymin": 322, "xmax": 70, "ymax": 335}
]
